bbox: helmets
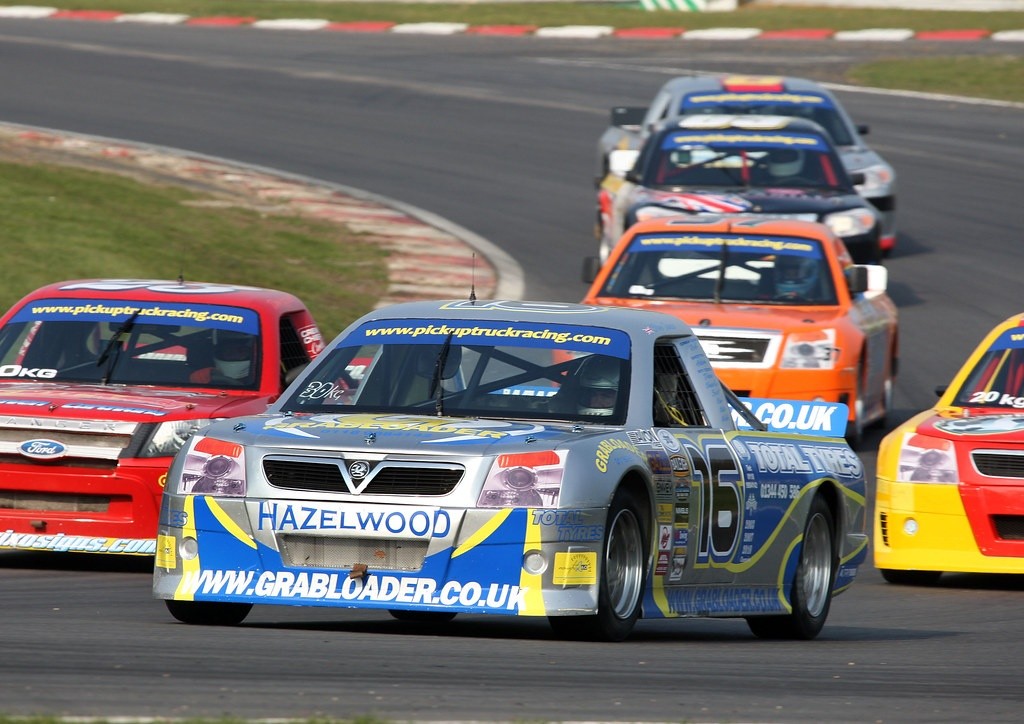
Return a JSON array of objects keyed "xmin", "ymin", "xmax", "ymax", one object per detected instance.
[
  {"xmin": 210, "ymin": 328, "xmax": 254, "ymax": 380},
  {"xmin": 772, "ymin": 254, "xmax": 820, "ymax": 297},
  {"xmin": 576, "ymin": 353, "xmax": 621, "ymax": 416},
  {"xmin": 767, "ymin": 148, "xmax": 806, "ymax": 177}
]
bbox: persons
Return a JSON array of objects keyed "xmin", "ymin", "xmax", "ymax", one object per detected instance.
[
  {"xmin": 190, "ymin": 323, "xmax": 257, "ymax": 387},
  {"xmin": 577, "ymin": 352, "xmax": 620, "ymax": 417},
  {"xmin": 776, "ymin": 145, "xmax": 811, "ymax": 186},
  {"xmin": 762, "ymin": 252, "xmax": 819, "ymax": 300}
]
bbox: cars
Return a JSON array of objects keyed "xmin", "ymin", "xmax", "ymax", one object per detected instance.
[
  {"xmin": 0, "ymin": 279, "xmax": 376, "ymax": 563},
  {"xmin": 600, "ymin": 72, "xmax": 900, "ymax": 251},
  {"xmin": 873, "ymin": 313, "xmax": 1024, "ymax": 589},
  {"xmin": 574, "ymin": 213, "xmax": 900, "ymax": 445},
  {"xmin": 147, "ymin": 301, "xmax": 869, "ymax": 642},
  {"xmin": 597, "ymin": 114, "xmax": 884, "ymax": 290}
]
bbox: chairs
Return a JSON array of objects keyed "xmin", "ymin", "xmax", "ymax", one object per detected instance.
[
  {"xmin": 665, "ymin": 166, "xmax": 738, "ymax": 185},
  {"xmin": 44, "ymin": 337, "xmax": 124, "ymax": 383},
  {"xmin": 756, "ymin": 266, "xmax": 823, "ymax": 299},
  {"xmin": 547, "ymin": 360, "xmax": 580, "ymax": 412},
  {"xmin": 622, "ymin": 271, "xmax": 700, "ymax": 297}
]
